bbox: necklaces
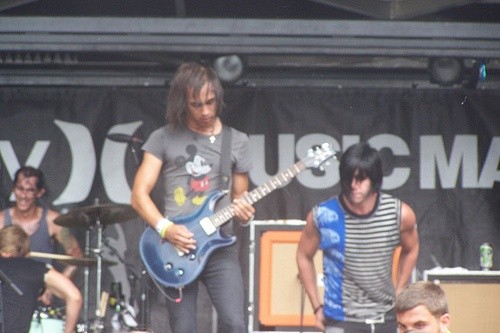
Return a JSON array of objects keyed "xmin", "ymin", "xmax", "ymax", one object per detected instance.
[{"xmin": 183, "ymin": 115, "xmax": 216, "ymax": 144}]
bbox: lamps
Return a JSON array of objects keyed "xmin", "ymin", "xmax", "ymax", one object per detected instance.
[
  {"xmin": 208, "ymin": 52, "xmax": 244, "ymax": 82},
  {"xmin": 427, "ymin": 56, "xmax": 464, "ymax": 87}
]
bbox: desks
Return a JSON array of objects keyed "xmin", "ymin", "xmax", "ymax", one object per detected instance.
[
  {"xmin": 424, "ymin": 271, "xmax": 500, "ymax": 333},
  {"xmin": 249, "ymin": 222, "xmax": 326, "ymax": 331}
]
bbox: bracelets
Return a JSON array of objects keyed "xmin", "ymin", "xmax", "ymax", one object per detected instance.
[
  {"xmin": 314, "ymin": 305, "xmax": 323, "ymax": 315},
  {"xmin": 240, "ymin": 215, "xmax": 254, "ymax": 227},
  {"xmin": 155, "ymin": 218, "xmax": 174, "ymax": 238}
]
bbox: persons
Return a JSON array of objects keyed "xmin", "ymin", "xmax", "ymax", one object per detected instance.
[
  {"xmin": 394, "ymin": 280, "xmax": 452, "ymax": 333},
  {"xmin": 131, "ymin": 62, "xmax": 256, "ymax": 333},
  {"xmin": 296, "ymin": 143, "xmax": 419, "ymax": 333},
  {"xmin": 0, "ymin": 224, "xmax": 83, "ymax": 333},
  {"xmin": 0, "ymin": 166, "xmax": 84, "ymax": 306}
]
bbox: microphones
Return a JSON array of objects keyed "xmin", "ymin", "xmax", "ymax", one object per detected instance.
[
  {"xmin": 109, "ymin": 134, "xmax": 143, "ymax": 145},
  {"xmin": 111, "ymin": 282, "xmax": 122, "ymax": 312}
]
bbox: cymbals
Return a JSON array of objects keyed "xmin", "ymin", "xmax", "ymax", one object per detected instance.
[
  {"xmin": 24, "ymin": 251, "xmax": 118, "ymax": 268},
  {"xmin": 52, "ymin": 202, "xmax": 138, "ymax": 229}
]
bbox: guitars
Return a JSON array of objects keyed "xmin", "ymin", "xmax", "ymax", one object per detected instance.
[{"xmin": 139, "ymin": 143, "xmax": 338, "ymax": 289}]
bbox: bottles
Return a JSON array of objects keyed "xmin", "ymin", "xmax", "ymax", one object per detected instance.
[{"xmin": 104, "ymin": 282, "xmax": 127, "ymax": 333}]
